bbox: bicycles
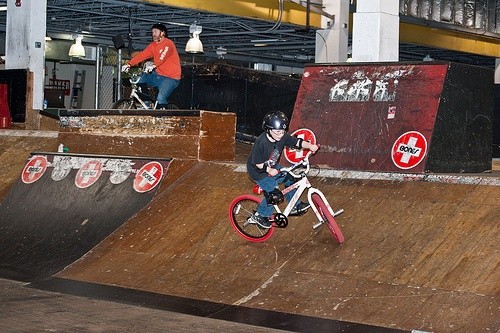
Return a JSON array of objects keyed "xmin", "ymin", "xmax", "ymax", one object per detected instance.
[
  {"xmin": 229, "ymin": 144, "xmax": 345, "ymax": 245},
  {"xmin": 110, "ymin": 66, "xmax": 181, "ymax": 110}
]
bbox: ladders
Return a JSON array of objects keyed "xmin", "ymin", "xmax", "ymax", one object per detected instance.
[{"xmin": 70, "ymin": 70, "xmax": 86, "ymax": 109}]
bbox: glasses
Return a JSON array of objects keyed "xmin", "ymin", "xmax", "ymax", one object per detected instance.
[{"xmin": 272, "ymin": 130, "xmax": 287, "ymax": 137}]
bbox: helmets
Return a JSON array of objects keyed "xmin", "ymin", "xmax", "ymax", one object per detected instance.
[{"xmin": 262, "ymin": 110, "xmax": 289, "ymax": 131}]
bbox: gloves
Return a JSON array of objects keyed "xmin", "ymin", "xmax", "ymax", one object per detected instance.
[
  {"xmin": 122, "ymin": 64, "xmax": 131, "ymax": 72},
  {"xmin": 145, "ymin": 65, "xmax": 156, "ymax": 73}
]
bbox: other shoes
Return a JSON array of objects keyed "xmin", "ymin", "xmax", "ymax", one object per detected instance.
[
  {"xmin": 290, "ymin": 202, "xmax": 311, "ymax": 214},
  {"xmin": 251, "ymin": 210, "xmax": 272, "ymax": 229}
]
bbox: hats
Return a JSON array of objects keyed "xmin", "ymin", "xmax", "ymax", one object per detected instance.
[{"xmin": 152, "ymin": 24, "xmax": 169, "ymax": 38}]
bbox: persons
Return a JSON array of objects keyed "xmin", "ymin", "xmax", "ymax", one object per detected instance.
[
  {"xmin": 122, "ymin": 25, "xmax": 181, "ymax": 109},
  {"xmin": 247, "ymin": 110, "xmax": 318, "ymax": 228}
]
